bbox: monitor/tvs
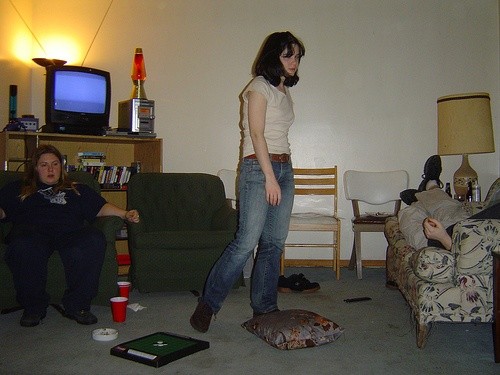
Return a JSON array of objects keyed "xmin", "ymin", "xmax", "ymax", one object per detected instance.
[{"xmin": 44, "ymin": 66, "xmax": 112, "ymax": 135}]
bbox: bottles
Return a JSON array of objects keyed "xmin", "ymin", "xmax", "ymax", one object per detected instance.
[
  {"xmin": 466, "ymin": 182, "xmax": 472, "ymax": 202},
  {"xmin": 445, "ymin": 183, "xmax": 452, "ymax": 199},
  {"xmin": 472, "ymin": 181, "xmax": 481, "ymax": 202}
]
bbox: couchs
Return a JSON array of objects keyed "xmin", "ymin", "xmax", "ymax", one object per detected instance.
[
  {"xmin": 125, "ymin": 173, "xmax": 245, "ymax": 289},
  {"xmin": 0, "ymin": 171, "xmax": 124, "ymax": 311},
  {"xmin": 280, "ymin": 166, "xmax": 341, "ymax": 280}
]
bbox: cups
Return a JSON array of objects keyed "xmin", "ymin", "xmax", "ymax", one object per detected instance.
[
  {"xmin": 110, "ymin": 297, "xmax": 128, "ymax": 323},
  {"xmin": 116, "ymin": 281, "xmax": 130, "ymax": 298}
]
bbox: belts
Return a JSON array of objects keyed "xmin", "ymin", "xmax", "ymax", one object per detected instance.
[{"xmin": 246, "ymin": 153, "xmax": 289, "ymax": 163}]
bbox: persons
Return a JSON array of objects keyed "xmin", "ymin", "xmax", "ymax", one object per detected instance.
[
  {"xmin": 400, "ymin": 155, "xmax": 500, "ymax": 252},
  {"xmin": 189, "ymin": 32, "xmax": 305, "ymax": 332},
  {"xmin": 0, "ymin": 146, "xmax": 140, "ymax": 326}
]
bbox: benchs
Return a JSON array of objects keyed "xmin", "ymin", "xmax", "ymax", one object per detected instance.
[{"xmin": 383, "ymin": 177, "xmax": 500, "ymax": 347}]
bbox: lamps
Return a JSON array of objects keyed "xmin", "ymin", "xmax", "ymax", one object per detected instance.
[{"xmin": 437, "ymin": 93, "xmax": 496, "ymax": 195}]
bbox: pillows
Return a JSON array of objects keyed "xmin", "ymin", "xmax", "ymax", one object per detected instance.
[{"xmin": 241, "ymin": 309, "xmax": 346, "ymax": 350}]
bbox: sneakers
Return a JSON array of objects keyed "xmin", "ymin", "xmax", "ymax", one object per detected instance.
[
  {"xmin": 190, "ymin": 297, "xmax": 216, "ymax": 333},
  {"xmin": 20, "ymin": 310, "xmax": 46, "ymax": 327},
  {"xmin": 400, "ymin": 189, "xmax": 422, "ymax": 206},
  {"xmin": 253, "ymin": 309, "xmax": 280, "ymax": 318},
  {"xmin": 419, "ymin": 155, "xmax": 444, "ymax": 192},
  {"xmin": 279, "ymin": 273, "xmax": 320, "ymax": 293},
  {"xmin": 386, "ymin": 281, "xmax": 399, "ymax": 289},
  {"xmin": 72, "ymin": 311, "xmax": 97, "ymax": 325}
]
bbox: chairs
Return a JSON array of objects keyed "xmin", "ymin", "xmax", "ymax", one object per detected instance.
[{"xmin": 344, "ymin": 170, "xmax": 410, "ymax": 278}]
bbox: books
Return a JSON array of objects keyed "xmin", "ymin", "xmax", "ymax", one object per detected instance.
[{"xmin": 66, "ymin": 152, "xmax": 134, "ymax": 186}]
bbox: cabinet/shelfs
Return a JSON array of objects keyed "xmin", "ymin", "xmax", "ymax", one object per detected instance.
[{"xmin": 0, "ymin": 131, "xmax": 163, "ymax": 276}]
